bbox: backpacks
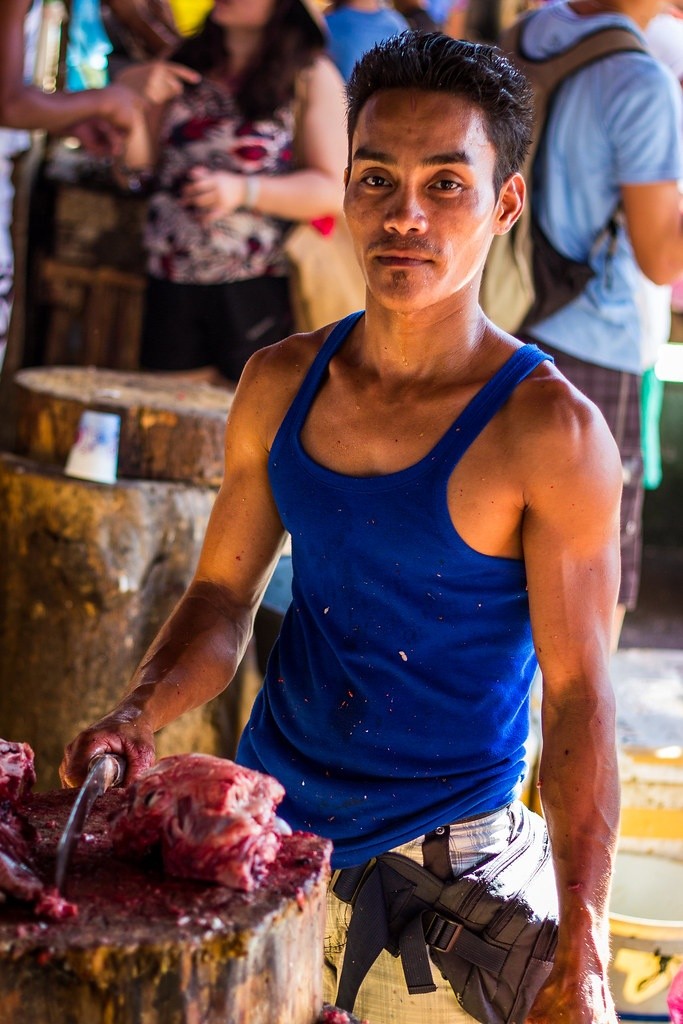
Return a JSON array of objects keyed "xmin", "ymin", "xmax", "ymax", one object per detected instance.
[{"xmin": 476, "ymin": 9, "xmax": 651, "ymax": 337}]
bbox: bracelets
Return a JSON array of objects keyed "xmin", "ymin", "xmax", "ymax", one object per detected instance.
[{"xmin": 244, "ymin": 174, "xmax": 261, "ymax": 211}]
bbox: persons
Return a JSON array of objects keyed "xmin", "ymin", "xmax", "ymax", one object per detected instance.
[
  {"xmin": 57, "ymin": 29, "xmax": 624, "ymax": 1024},
  {"xmin": 0, "ymin": 0, "xmax": 683, "ymax": 817}
]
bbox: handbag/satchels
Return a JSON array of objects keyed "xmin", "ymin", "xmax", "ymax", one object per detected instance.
[
  {"xmin": 265, "ymin": 207, "xmax": 367, "ymax": 335},
  {"xmin": 327, "ymin": 801, "xmax": 559, "ymax": 1024}
]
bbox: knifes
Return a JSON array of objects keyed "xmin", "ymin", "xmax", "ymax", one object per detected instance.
[{"xmin": 49, "ymin": 757, "xmax": 124, "ymax": 890}]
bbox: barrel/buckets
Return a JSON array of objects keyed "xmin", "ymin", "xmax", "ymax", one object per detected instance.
[{"xmin": 607, "ymin": 849, "xmax": 683, "ymax": 1024}]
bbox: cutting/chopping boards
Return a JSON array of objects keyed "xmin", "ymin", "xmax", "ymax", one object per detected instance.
[
  {"xmin": 12, "ymin": 363, "xmax": 243, "ymax": 488},
  {"xmin": 0, "ymin": 834, "xmax": 333, "ymax": 1024}
]
